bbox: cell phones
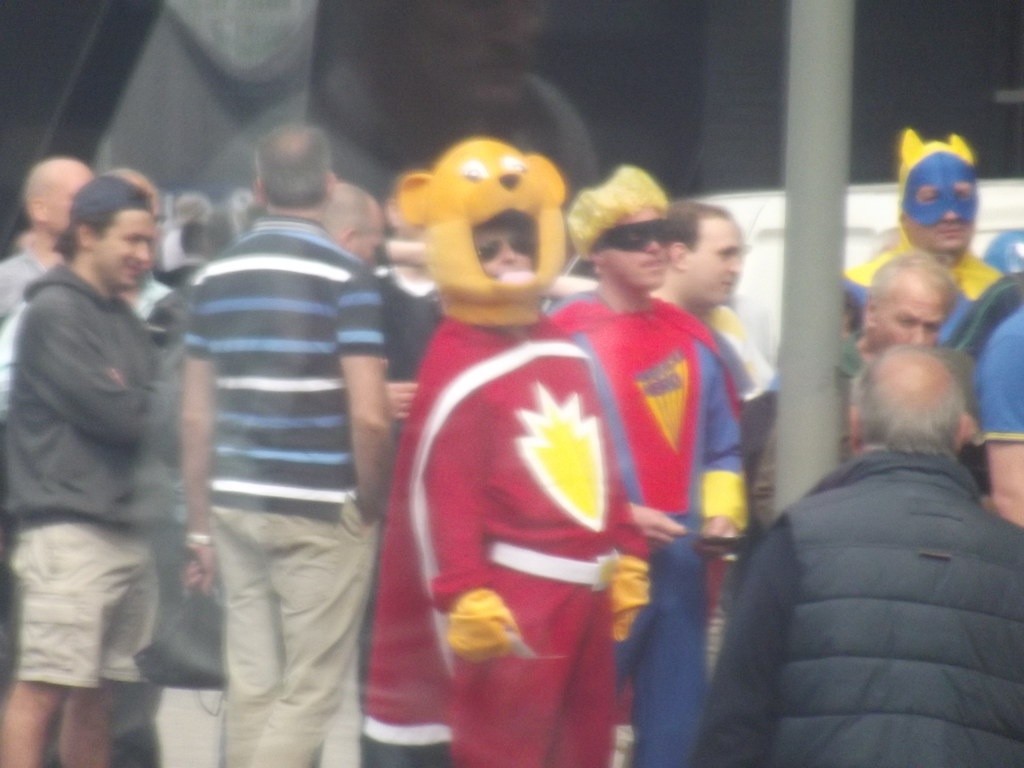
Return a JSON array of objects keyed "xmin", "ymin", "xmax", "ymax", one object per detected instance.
[{"xmin": 697, "ymin": 534, "xmax": 747, "ymax": 545}]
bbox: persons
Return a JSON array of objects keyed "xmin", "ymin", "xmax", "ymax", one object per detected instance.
[
  {"xmin": 694, "ymin": 344, "xmax": 1024, "ymax": 768},
  {"xmin": 547, "ymin": 165, "xmax": 772, "ymax": 767},
  {"xmin": 363, "ymin": 138, "xmax": 651, "ymax": 767},
  {"xmin": 177, "ymin": 124, "xmax": 394, "ymax": 768},
  {"xmin": 3, "ymin": 176, "xmax": 178, "ymax": 766},
  {"xmin": 0, "ymin": 129, "xmax": 1022, "ymax": 526}
]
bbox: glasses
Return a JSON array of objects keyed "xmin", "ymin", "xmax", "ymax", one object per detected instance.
[
  {"xmin": 593, "ymin": 219, "xmax": 669, "ymax": 252},
  {"xmin": 476, "ymin": 233, "xmax": 534, "ymax": 264}
]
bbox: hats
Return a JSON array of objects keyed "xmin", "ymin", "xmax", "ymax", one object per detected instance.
[{"xmin": 50, "ymin": 177, "xmax": 147, "ymax": 252}]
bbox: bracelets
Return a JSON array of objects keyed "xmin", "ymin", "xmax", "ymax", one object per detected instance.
[{"xmin": 182, "ymin": 534, "xmax": 211, "ymax": 545}]
